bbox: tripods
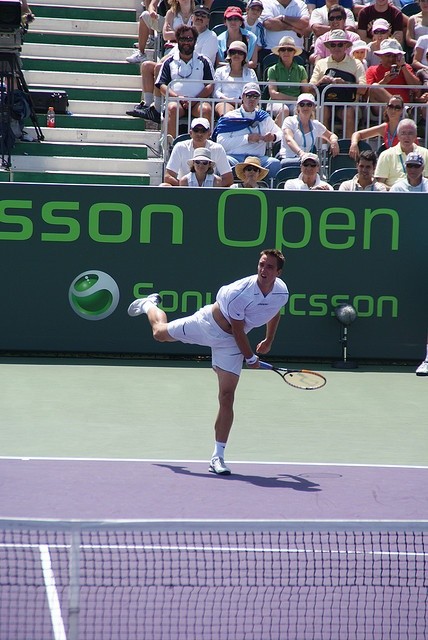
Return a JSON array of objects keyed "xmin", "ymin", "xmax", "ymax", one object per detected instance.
[{"xmin": 1, "ymin": 53, "xmax": 45, "ymax": 172}]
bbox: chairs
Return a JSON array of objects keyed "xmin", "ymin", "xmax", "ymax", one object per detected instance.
[
  {"xmin": 273, "ymin": 166, "xmax": 301, "ymax": 189},
  {"xmin": 262, "ymin": 53, "xmax": 306, "ymax": 82},
  {"xmin": 328, "ymin": 168, "xmax": 359, "ymax": 190},
  {"xmin": 328, "ymin": 138, "xmax": 373, "ymax": 174}
]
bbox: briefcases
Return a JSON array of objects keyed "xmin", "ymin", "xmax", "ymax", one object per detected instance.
[{"xmin": 23, "ymin": 87, "xmax": 69, "ymax": 114}]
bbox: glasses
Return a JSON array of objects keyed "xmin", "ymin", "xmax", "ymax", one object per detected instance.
[
  {"xmin": 278, "ymin": 47, "xmax": 294, "ymax": 52},
  {"xmin": 194, "ymin": 159, "xmax": 209, "ymax": 166},
  {"xmin": 193, "ymin": 11, "xmax": 207, "ymax": 18},
  {"xmin": 405, "ymin": 163, "xmax": 421, "ymax": 168},
  {"xmin": 229, "ymin": 49, "xmax": 243, "ymax": 56},
  {"xmin": 329, "ymin": 42, "xmax": 344, "ymax": 48},
  {"xmin": 371, "ymin": 28, "xmax": 388, "ymax": 34},
  {"xmin": 386, "ymin": 104, "xmax": 402, "ymax": 111},
  {"xmin": 243, "ymin": 92, "xmax": 259, "ymax": 98},
  {"xmin": 191, "ymin": 126, "xmax": 207, "ymax": 134},
  {"xmin": 177, "ymin": 35, "xmax": 195, "ymax": 43},
  {"xmin": 226, "ymin": 16, "xmax": 240, "ymax": 22},
  {"xmin": 327, "ymin": 15, "xmax": 344, "ymax": 22},
  {"xmin": 301, "ymin": 162, "xmax": 318, "ymax": 168},
  {"xmin": 244, "ymin": 166, "xmax": 259, "ymax": 172},
  {"xmin": 397, "ymin": 132, "xmax": 416, "ymax": 137},
  {"xmin": 250, "ymin": 6, "xmax": 261, "ymax": 13},
  {"xmin": 297, "ymin": 102, "xmax": 313, "ymax": 107}
]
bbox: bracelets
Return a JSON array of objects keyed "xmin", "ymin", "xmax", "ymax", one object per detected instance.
[{"xmin": 245, "ymin": 353, "xmax": 257, "ymax": 365}]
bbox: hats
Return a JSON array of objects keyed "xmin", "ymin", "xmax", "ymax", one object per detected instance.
[
  {"xmin": 300, "ymin": 152, "xmax": 319, "ymax": 164},
  {"xmin": 191, "ymin": 117, "xmax": 210, "ymax": 130},
  {"xmin": 403, "ymin": 152, "xmax": 423, "ymax": 166},
  {"xmin": 226, "ymin": 40, "xmax": 247, "ymax": 57},
  {"xmin": 297, "ymin": 93, "xmax": 314, "ymax": 104},
  {"xmin": 223, "ymin": 6, "xmax": 243, "ymax": 20},
  {"xmin": 234, "ymin": 156, "xmax": 269, "ymax": 182},
  {"xmin": 241, "ymin": 83, "xmax": 261, "ymax": 96},
  {"xmin": 321, "ymin": 29, "xmax": 352, "ymax": 48},
  {"xmin": 371, "ymin": 18, "xmax": 389, "ymax": 32},
  {"xmin": 270, "ymin": 35, "xmax": 302, "ymax": 57},
  {"xmin": 348, "ymin": 41, "xmax": 370, "ymax": 58},
  {"xmin": 191, "ymin": 147, "xmax": 215, "ymax": 168},
  {"xmin": 371, "ymin": 38, "xmax": 405, "ymax": 56},
  {"xmin": 246, "ymin": 0, "xmax": 262, "ymax": 10},
  {"xmin": 193, "ymin": 3, "xmax": 210, "ymax": 14}
]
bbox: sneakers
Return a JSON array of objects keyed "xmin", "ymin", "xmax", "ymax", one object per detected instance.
[
  {"xmin": 126, "ymin": 100, "xmax": 149, "ymax": 117},
  {"xmin": 134, "ymin": 36, "xmax": 154, "ymax": 48},
  {"xmin": 125, "ymin": 52, "xmax": 148, "ymax": 65},
  {"xmin": 134, "ymin": 104, "xmax": 160, "ymax": 123},
  {"xmin": 208, "ymin": 456, "xmax": 230, "ymax": 475},
  {"xmin": 415, "ymin": 361, "xmax": 427, "ymax": 375},
  {"xmin": 127, "ymin": 292, "xmax": 162, "ymax": 317}
]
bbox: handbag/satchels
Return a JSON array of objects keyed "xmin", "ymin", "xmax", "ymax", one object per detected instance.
[{"xmin": 318, "ymin": 70, "xmax": 358, "ymax": 102}]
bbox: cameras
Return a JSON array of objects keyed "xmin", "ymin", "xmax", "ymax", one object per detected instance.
[{"xmin": 390, "ymin": 64, "xmax": 399, "ymax": 74}]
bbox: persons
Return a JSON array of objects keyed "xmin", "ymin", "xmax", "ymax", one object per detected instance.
[
  {"xmin": 127, "ymin": 248, "xmax": 289, "ymax": 476},
  {"xmin": 0, "ymin": 0, "xmax": 35, "ymax": 143},
  {"xmin": 125, "ymin": 0, "xmax": 427, "ymax": 192}
]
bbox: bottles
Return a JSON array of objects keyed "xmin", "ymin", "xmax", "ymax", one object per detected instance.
[{"xmin": 47, "ymin": 107, "xmax": 55, "ymax": 127}]
